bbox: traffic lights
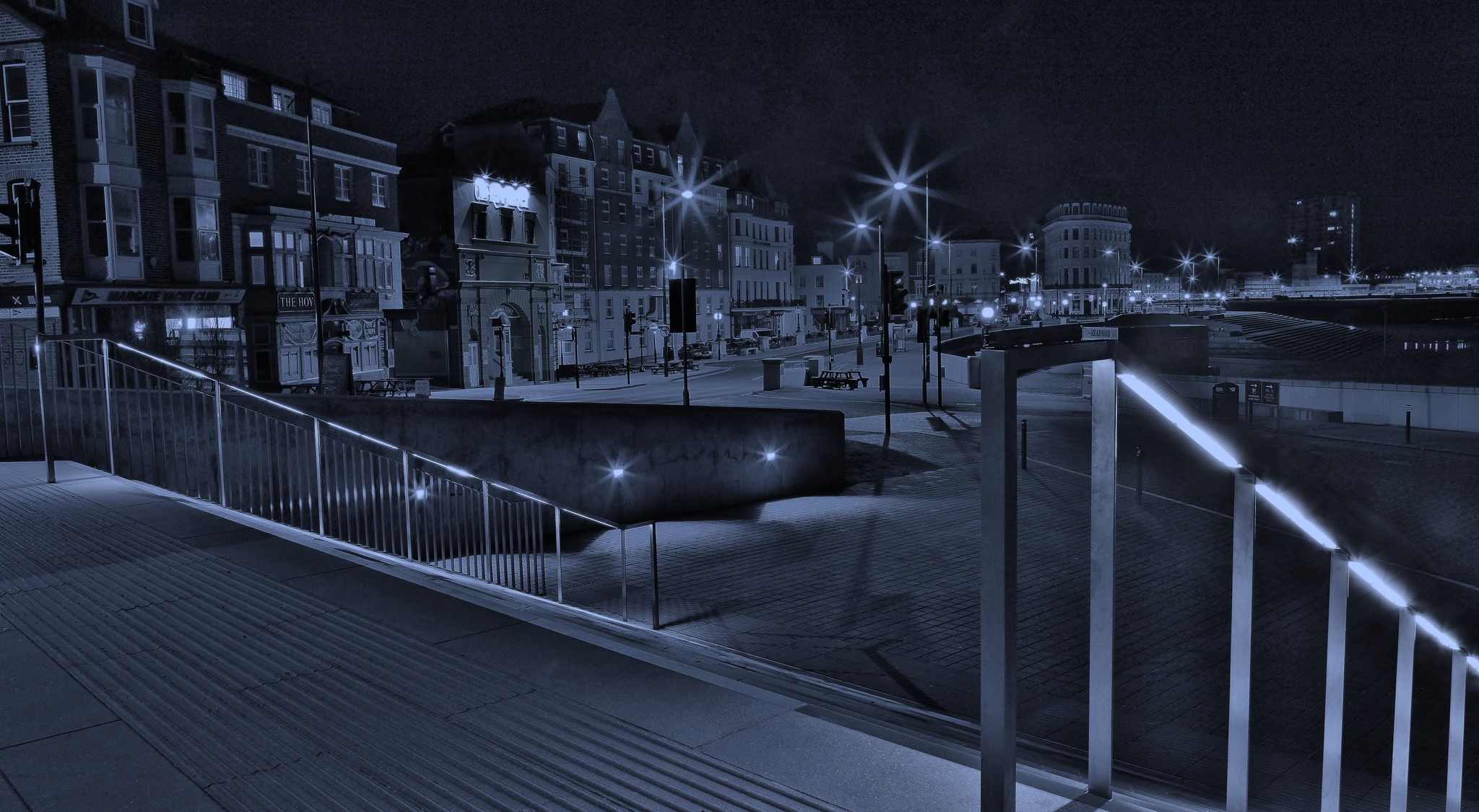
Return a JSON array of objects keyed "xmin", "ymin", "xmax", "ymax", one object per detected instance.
[
  {"xmin": 938, "ymin": 295, "xmax": 951, "ymax": 328},
  {"xmin": 924, "ymin": 295, "xmax": 939, "ymax": 319},
  {"xmin": 625, "ymin": 311, "xmax": 637, "ymax": 332},
  {"xmin": 0, "ymin": 200, "xmax": 21, "ymax": 263},
  {"xmin": 887, "ymin": 268, "xmax": 909, "ymax": 317}
]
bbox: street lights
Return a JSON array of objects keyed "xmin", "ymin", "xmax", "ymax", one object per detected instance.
[
  {"xmin": 658, "ymin": 190, "xmax": 695, "ymax": 361},
  {"xmin": 844, "ymin": 269, "xmax": 861, "ymax": 337},
  {"xmin": 1129, "ymin": 296, "xmax": 1135, "ymax": 312},
  {"xmin": 1053, "ymin": 300, "xmax": 1056, "ymax": 319},
  {"xmin": 896, "ymin": 179, "xmax": 931, "ymax": 295},
  {"xmin": 714, "ymin": 311, "xmax": 723, "ymax": 342},
  {"xmin": 1106, "ymin": 250, "xmax": 1121, "ymax": 313},
  {"xmin": 851, "ymin": 217, "xmax": 893, "ymax": 435},
  {"xmin": 795, "ymin": 303, "xmax": 802, "ymax": 332},
  {"xmin": 933, "ymin": 239, "xmax": 953, "ymax": 298},
  {"xmin": 1102, "ymin": 282, "xmax": 1107, "ymax": 316},
  {"xmin": 1024, "ymin": 245, "xmax": 1039, "ymax": 309},
  {"xmin": 714, "ymin": 307, "xmax": 724, "ymax": 362},
  {"xmin": 1146, "ymin": 284, "xmax": 1150, "ymax": 311},
  {"xmin": 567, "ymin": 325, "xmax": 581, "ymax": 389}
]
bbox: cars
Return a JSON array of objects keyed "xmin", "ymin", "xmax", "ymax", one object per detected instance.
[
  {"xmin": 673, "ymin": 326, "xmax": 781, "ymax": 360},
  {"xmin": 863, "ymin": 313, "xmax": 909, "ymax": 326},
  {"xmin": 973, "ymin": 313, "xmax": 1047, "ymax": 330}
]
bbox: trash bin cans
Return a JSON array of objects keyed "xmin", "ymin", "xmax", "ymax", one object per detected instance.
[
  {"xmin": 1210, "ymin": 382, "xmax": 1240, "ymax": 431},
  {"xmin": 876, "ymin": 342, "xmax": 882, "ymax": 358}
]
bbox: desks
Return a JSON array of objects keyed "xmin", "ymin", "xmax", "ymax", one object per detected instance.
[
  {"xmin": 278, "ymin": 380, "xmax": 319, "ymax": 394},
  {"xmin": 357, "ymin": 374, "xmax": 435, "ymax": 398},
  {"xmin": 577, "ymin": 360, "xmax": 701, "ymax": 380},
  {"xmin": 812, "ymin": 368, "xmax": 869, "ymax": 390}
]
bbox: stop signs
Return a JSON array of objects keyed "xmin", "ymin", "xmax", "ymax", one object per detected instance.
[{"xmin": 648, "ymin": 323, "xmax": 658, "ymax": 333}]
